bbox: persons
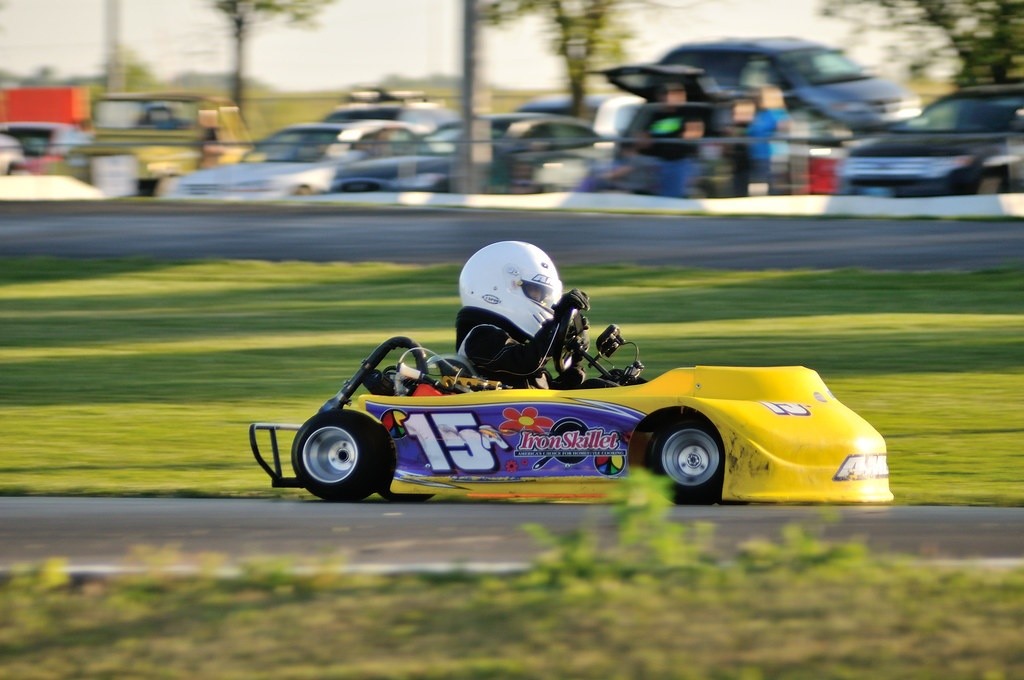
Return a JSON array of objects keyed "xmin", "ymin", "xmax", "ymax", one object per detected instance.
[
  {"xmin": 582, "ymin": 85, "xmax": 793, "ymax": 199},
  {"xmin": 456, "ymin": 241, "xmax": 647, "ymax": 389}
]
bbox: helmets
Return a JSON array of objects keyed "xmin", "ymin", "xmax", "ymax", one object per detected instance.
[{"xmin": 459, "ymin": 241, "xmax": 564, "ymax": 339}]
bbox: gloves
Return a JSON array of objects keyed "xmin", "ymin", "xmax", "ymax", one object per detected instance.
[
  {"xmin": 551, "ymin": 288, "xmax": 591, "ymax": 323},
  {"xmin": 570, "ymin": 320, "xmax": 591, "ymax": 353}
]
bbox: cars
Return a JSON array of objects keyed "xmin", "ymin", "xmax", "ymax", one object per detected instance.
[
  {"xmin": 834, "ymin": 82, "xmax": 1024, "ymax": 200},
  {"xmin": 0, "ymin": 35, "xmax": 917, "ymax": 199}
]
